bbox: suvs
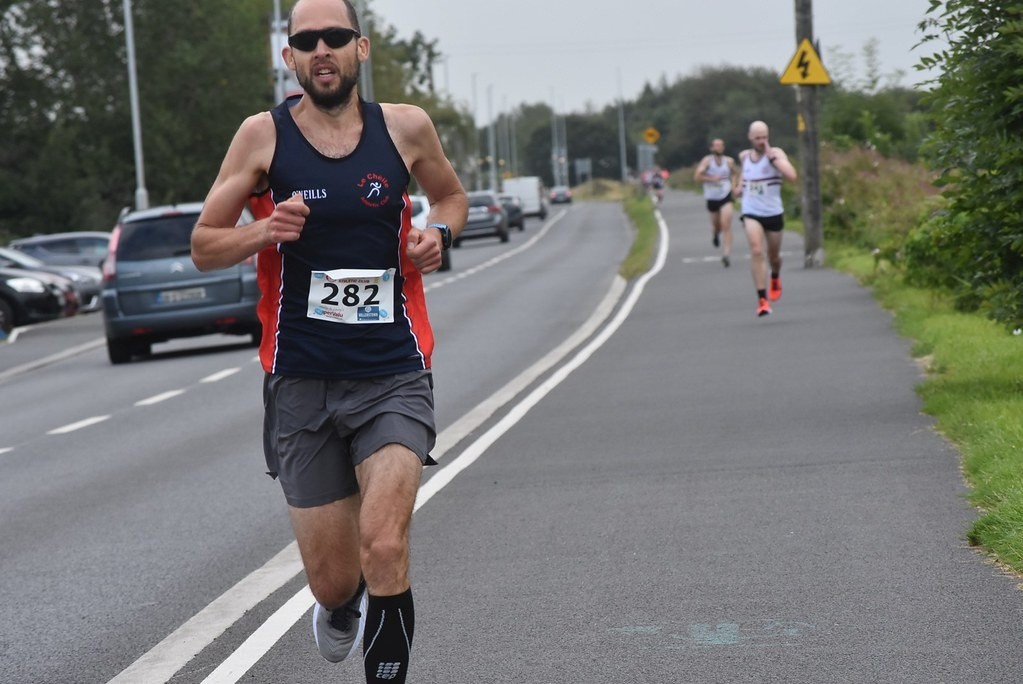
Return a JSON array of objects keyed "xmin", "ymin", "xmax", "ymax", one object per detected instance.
[
  {"xmin": 449, "ymin": 189, "xmax": 512, "ymax": 250},
  {"xmin": 99, "ymin": 202, "xmax": 265, "ymax": 365},
  {"xmin": 407, "ymin": 192, "xmax": 451, "ymax": 275}
]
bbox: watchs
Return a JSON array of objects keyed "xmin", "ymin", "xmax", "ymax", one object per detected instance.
[{"xmin": 429, "ymin": 224, "xmax": 452, "ymax": 250}]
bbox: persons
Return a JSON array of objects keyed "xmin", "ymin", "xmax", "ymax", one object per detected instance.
[
  {"xmin": 694, "ymin": 138, "xmax": 737, "ymax": 266},
  {"xmin": 189, "ymin": 0, "xmax": 470, "ymax": 684},
  {"xmin": 640, "ymin": 165, "xmax": 668, "ymax": 205},
  {"xmin": 734, "ymin": 120, "xmax": 797, "ymax": 318}
]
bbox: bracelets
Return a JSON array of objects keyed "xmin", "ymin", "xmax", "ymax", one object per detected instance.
[{"xmin": 770, "ymin": 157, "xmax": 776, "ymax": 163}]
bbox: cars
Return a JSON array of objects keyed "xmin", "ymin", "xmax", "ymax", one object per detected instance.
[
  {"xmin": 545, "ymin": 183, "xmax": 573, "ymax": 205},
  {"xmin": 497, "ymin": 192, "xmax": 527, "ymax": 232},
  {"xmin": 0, "ymin": 231, "xmax": 114, "ymax": 340}
]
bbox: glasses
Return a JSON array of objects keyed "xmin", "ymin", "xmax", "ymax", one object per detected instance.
[{"xmin": 288, "ymin": 27, "xmax": 361, "ymax": 52}]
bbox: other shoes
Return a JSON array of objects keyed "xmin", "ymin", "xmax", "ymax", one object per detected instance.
[
  {"xmin": 712, "ymin": 233, "xmax": 720, "ymax": 247},
  {"xmin": 721, "ymin": 256, "xmax": 731, "ymax": 266}
]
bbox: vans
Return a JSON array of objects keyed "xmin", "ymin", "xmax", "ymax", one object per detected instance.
[{"xmin": 500, "ymin": 174, "xmax": 547, "ymax": 220}]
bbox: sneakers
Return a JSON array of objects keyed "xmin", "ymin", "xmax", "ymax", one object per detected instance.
[
  {"xmin": 312, "ymin": 572, "xmax": 368, "ymax": 664},
  {"xmin": 768, "ymin": 278, "xmax": 782, "ymax": 300},
  {"xmin": 756, "ymin": 297, "xmax": 771, "ymax": 319}
]
